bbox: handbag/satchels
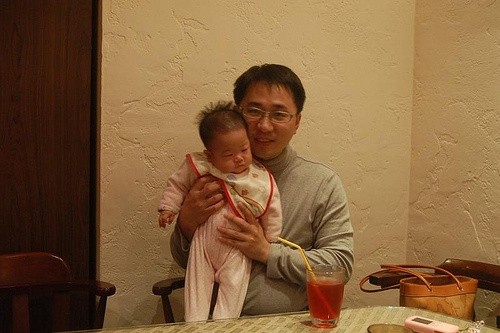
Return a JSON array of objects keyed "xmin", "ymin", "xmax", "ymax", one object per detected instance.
[{"xmin": 358, "ymin": 263, "xmax": 479, "ymax": 322}]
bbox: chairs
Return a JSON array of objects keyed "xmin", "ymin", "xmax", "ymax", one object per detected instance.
[
  {"xmin": 153, "ymin": 277, "xmax": 185, "ymax": 324},
  {"xmin": 0, "ymin": 252, "xmax": 116, "ymax": 333},
  {"xmin": 435, "ymin": 258, "xmax": 500, "ymax": 330}
]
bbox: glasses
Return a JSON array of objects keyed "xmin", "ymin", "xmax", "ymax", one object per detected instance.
[{"xmin": 239, "ymin": 105, "xmax": 298, "ymax": 123}]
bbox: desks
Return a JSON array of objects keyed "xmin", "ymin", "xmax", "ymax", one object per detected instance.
[{"xmin": 79, "ymin": 306, "xmax": 500, "ymax": 333}]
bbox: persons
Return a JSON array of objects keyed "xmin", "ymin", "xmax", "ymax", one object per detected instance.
[
  {"xmin": 169, "ymin": 64, "xmax": 355, "ymax": 318},
  {"xmin": 157, "ymin": 100, "xmax": 284, "ymax": 323}
]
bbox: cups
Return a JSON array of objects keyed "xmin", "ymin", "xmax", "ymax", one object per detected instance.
[{"xmin": 306, "ymin": 263, "xmax": 345, "ymax": 328}]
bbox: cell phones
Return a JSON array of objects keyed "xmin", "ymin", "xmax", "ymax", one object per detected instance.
[{"xmin": 404, "ymin": 315, "xmax": 459, "ymax": 333}]
